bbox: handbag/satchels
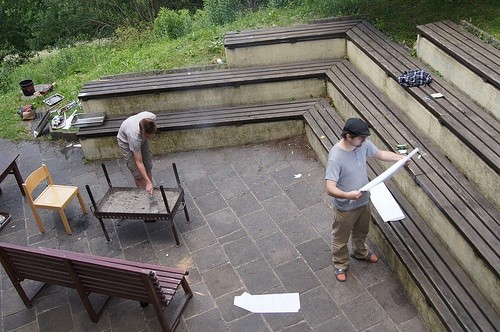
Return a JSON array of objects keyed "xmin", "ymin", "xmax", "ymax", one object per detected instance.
[{"xmin": 397, "ymin": 69, "xmax": 433, "ymax": 88}]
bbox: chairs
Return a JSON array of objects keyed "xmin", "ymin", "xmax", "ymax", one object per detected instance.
[{"xmin": 23, "ymin": 157, "xmax": 88, "ymax": 235}]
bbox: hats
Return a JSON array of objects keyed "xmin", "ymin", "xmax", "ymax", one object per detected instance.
[{"xmin": 344, "ymin": 118, "xmax": 371, "ymax": 136}]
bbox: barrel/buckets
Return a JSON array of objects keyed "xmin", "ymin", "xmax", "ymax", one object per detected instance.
[{"xmin": 19, "ymin": 79, "xmax": 35, "ymax": 96}]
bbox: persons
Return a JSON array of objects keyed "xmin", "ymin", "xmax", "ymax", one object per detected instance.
[
  {"xmin": 117, "ymin": 110, "xmax": 158, "ymax": 222},
  {"xmin": 324, "ymin": 118, "xmax": 411, "ymax": 281}
]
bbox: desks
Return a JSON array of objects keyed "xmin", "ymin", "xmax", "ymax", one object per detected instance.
[{"xmin": 0, "ymin": 138, "xmax": 28, "ymax": 197}]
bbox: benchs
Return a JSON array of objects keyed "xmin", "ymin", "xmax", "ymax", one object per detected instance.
[{"xmin": 0, "ymin": 242, "xmax": 495, "ymax": 323}]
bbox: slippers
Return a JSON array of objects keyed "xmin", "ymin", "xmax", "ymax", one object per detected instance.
[
  {"xmin": 334, "ymin": 267, "xmax": 348, "ymax": 281},
  {"xmin": 350, "ymin": 252, "xmax": 377, "ymax": 262}
]
